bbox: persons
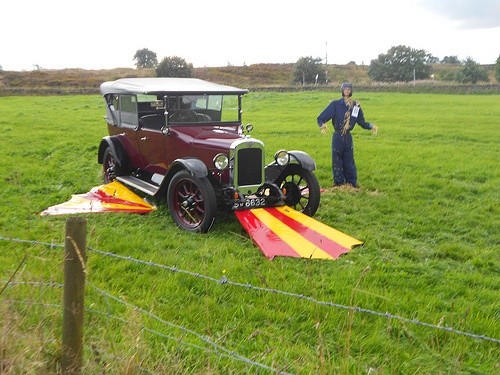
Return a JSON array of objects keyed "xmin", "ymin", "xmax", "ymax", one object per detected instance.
[{"xmin": 317, "ymin": 81, "xmax": 378, "ymax": 188}]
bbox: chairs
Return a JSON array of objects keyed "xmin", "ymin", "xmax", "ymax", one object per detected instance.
[
  {"xmin": 139, "ymin": 114, "xmax": 164, "ymax": 130},
  {"xmin": 124, "ymin": 102, "xmax": 185, "ymax": 114}
]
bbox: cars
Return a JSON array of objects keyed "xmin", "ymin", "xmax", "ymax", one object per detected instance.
[{"xmin": 97, "ymin": 77, "xmax": 321, "ymax": 234}]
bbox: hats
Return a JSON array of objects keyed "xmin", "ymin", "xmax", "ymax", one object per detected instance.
[{"xmin": 342, "ymin": 83, "xmax": 352, "ymax": 96}]
